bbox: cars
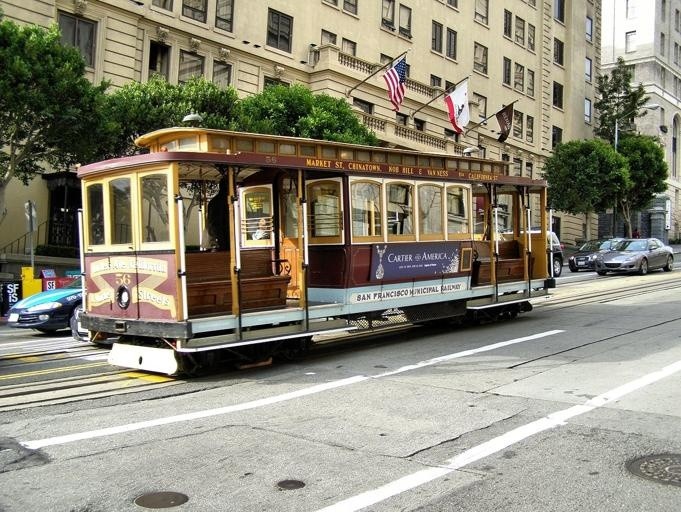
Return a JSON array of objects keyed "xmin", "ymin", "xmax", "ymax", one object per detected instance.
[
  {"xmin": 568, "ymin": 237, "xmax": 674, "ymax": 276},
  {"xmin": 547, "ymin": 231, "xmax": 565, "ymax": 277},
  {"xmin": 7, "ymin": 277, "xmax": 121, "ymax": 346}
]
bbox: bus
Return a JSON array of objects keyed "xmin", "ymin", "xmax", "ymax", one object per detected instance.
[{"xmin": 71, "ymin": 113, "xmax": 555, "ymax": 379}]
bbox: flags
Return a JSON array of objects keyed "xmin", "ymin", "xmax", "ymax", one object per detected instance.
[
  {"xmin": 445, "ymin": 81, "xmax": 469, "ymax": 133},
  {"xmin": 383, "ymin": 55, "xmax": 406, "ymax": 112},
  {"xmin": 496, "ymin": 103, "xmax": 513, "ymax": 143}
]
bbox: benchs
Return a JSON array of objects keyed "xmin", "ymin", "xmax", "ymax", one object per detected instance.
[
  {"xmin": 185, "ymin": 248, "xmax": 291, "ymax": 318},
  {"xmin": 472, "ymin": 239, "xmax": 535, "ymax": 285}
]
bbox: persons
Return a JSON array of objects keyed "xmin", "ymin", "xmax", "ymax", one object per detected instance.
[{"xmin": 208, "ymin": 176, "xmax": 229, "ymax": 251}]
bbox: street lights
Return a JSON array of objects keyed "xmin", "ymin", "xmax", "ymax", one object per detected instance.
[{"xmin": 612, "ymin": 104, "xmax": 660, "ymax": 237}]
